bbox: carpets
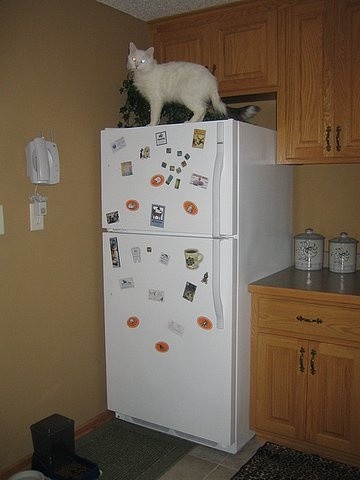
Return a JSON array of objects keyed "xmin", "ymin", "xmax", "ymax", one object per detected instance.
[
  {"xmin": 228, "ymin": 440, "xmax": 360, "ymax": 480},
  {"xmin": 73, "ymin": 415, "xmax": 198, "ymax": 480}
]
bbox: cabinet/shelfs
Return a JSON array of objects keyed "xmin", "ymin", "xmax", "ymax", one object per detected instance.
[
  {"xmin": 144, "ymin": 0, "xmax": 278, "ymax": 97},
  {"xmin": 246, "ymin": 261, "xmax": 360, "ymax": 469},
  {"xmin": 272, "ymin": 0, "xmax": 360, "ymax": 166}
]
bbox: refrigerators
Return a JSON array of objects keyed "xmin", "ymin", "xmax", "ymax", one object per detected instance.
[{"xmin": 98, "ymin": 119, "xmax": 296, "ymax": 456}]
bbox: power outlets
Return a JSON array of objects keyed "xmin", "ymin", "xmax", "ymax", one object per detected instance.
[{"xmin": 28, "ymin": 203, "xmax": 45, "ymax": 232}]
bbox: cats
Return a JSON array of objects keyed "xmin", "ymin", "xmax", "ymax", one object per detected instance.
[{"xmin": 125, "ymin": 39, "xmax": 261, "ymax": 127}]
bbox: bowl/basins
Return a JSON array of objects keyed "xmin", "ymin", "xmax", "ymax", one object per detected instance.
[{"xmin": 9, "ymin": 469, "xmax": 45, "ymax": 480}]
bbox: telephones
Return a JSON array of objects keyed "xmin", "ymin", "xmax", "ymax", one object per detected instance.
[{"xmin": 25, "ymin": 130, "xmax": 60, "ymax": 202}]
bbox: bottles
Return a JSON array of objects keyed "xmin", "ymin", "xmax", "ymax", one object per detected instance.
[
  {"xmin": 293, "ymin": 227, "xmax": 326, "ymax": 271},
  {"xmin": 328, "ymin": 231, "xmax": 357, "ymax": 274}
]
